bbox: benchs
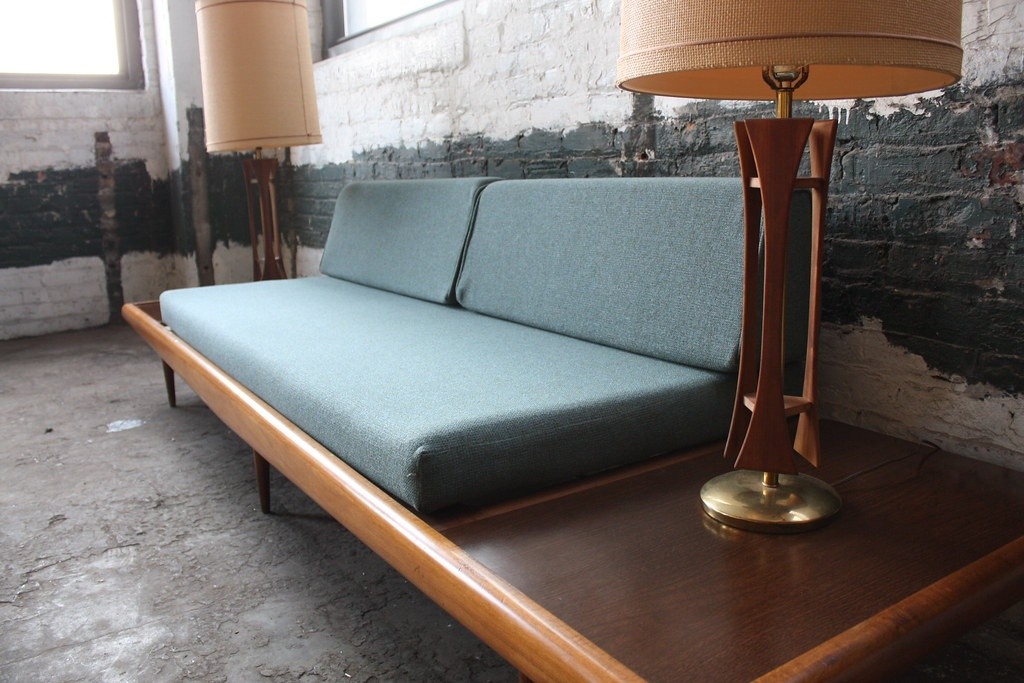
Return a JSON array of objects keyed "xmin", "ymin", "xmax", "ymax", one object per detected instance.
[{"xmin": 121, "ymin": 176, "xmax": 1024, "ymax": 683}]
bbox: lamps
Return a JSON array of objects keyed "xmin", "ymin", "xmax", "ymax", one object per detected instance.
[
  {"xmin": 614, "ymin": 0, "xmax": 964, "ymax": 533},
  {"xmin": 194, "ymin": 0, "xmax": 322, "ymax": 282}
]
